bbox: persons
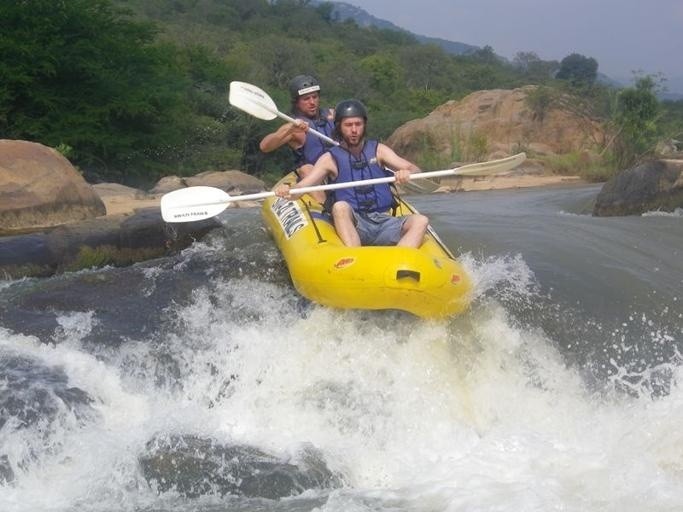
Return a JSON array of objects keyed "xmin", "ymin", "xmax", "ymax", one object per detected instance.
[
  {"xmin": 273, "ymin": 97, "xmax": 429, "ymax": 247},
  {"xmin": 257, "ymin": 73, "xmax": 337, "ymax": 205}
]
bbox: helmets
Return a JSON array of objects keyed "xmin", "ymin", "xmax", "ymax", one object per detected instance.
[
  {"xmin": 287, "ymin": 74, "xmax": 322, "ymax": 99},
  {"xmin": 331, "ymin": 99, "xmax": 367, "ymax": 124}
]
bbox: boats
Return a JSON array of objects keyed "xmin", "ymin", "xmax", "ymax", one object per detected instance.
[{"xmin": 262, "ymin": 171, "xmax": 473, "ymax": 326}]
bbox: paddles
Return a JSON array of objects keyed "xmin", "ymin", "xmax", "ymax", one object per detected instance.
[
  {"xmin": 228, "ymin": 81, "xmax": 440, "ymax": 195},
  {"xmin": 161, "ymin": 151, "xmax": 526, "ymax": 223}
]
